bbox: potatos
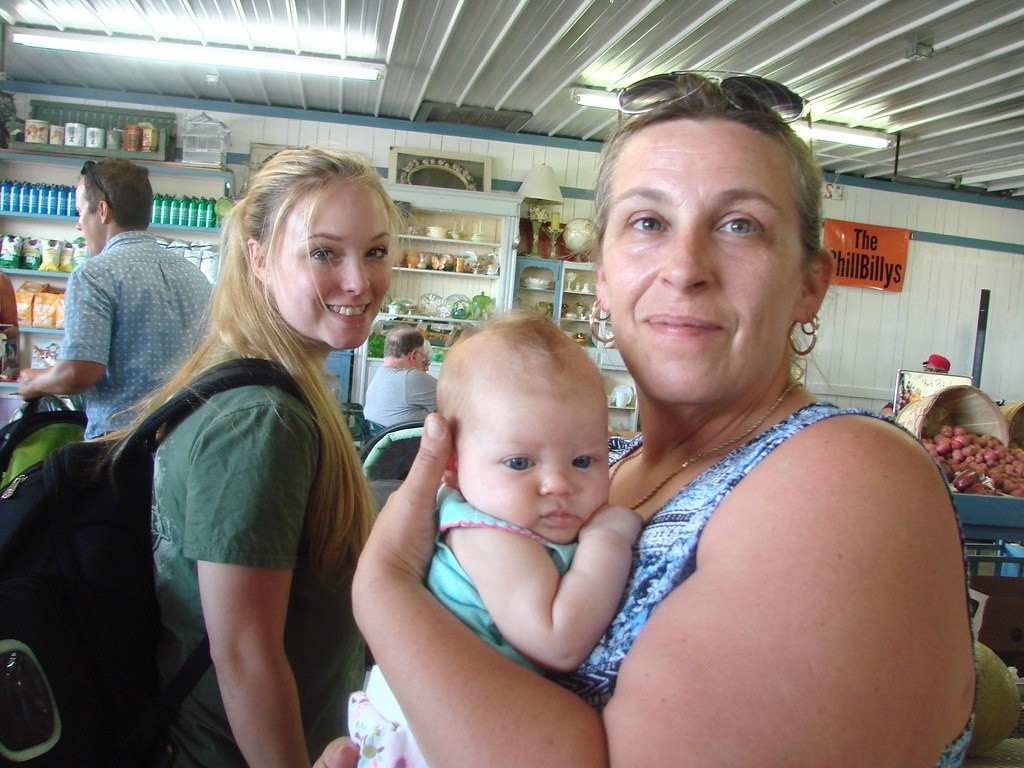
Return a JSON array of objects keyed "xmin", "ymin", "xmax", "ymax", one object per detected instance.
[{"xmin": 920, "ymin": 424, "xmax": 1024, "ymax": 498}]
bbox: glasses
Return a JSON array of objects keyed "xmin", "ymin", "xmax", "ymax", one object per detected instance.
[
  {"xmin": 81, "ymin": 160, "xmax": 113, "ymax": 210},
  {"xmin": 615, "ymin": 70, "xmax": 813, "ymax": 156},
  {"xmin": 410, "ymin": 350, "xmax": 432, "ymax": 366},
  {"xmin": 924, "ymin": 366, "xmax": 944, "ymax": 373}
]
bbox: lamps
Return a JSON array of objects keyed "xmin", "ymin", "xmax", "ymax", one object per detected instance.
[
  {"xmin": 8, "ymin": 24, "xmax": 388, "ymax": 81},
  {"xmin": 571, "ymin": 87, "xmax": 896, "ymax": 151},
  {"xmin": 516, "ymin": 162, "xmax": 564, "ymax": 258}
]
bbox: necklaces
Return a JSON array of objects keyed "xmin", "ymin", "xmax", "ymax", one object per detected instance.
[{"xmin": 608, "ymin": 382, "xmax": 804, "ymax": 510}]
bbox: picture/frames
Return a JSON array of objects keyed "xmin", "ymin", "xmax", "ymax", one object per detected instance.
[{"xmin": 387, "ymin": 145, "xmax": 492, "ymax": 192}]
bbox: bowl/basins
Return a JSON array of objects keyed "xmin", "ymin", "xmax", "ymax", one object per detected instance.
[
  {"xmin": 607, "ymin": 396, "xmax": 613, "ymax": 405},
  {"xmin": 427, "ymin": 225, "xmax": 447, "ymax": 237}
]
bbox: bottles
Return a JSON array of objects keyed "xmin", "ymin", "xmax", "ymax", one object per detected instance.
[
  {"xmin": 0, "ymin": 179, "xmax": 209, "ymax": 227},
  {"xmin": 125, "ymin": 125, "xmax": 139, "ymax": 151},
  {"xmin": 139, "ymin": 121, "xmax": 157, "ymax": 152}
]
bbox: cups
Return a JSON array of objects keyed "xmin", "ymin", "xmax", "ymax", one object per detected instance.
[
  {"xmin": 617, "ymin": 393, "xmax": 631, "ymax": 406},
  {"xmin": 49, "ymin": 125, "xmax": 64, "ymax": 146},
  {"xmin": 107, "ymin": 127, "xmax": 122, "ymax": 149},
  {"xmin": 24, "ymin": 119, "xmax": 49, "ymax": 145},
  {"xmin": 65, "ymin": 122, "xmax": 86, "ymax": 147},
  {"xmin": 86, "ymin": 127, "xmax": 105, "ymax": 147}
]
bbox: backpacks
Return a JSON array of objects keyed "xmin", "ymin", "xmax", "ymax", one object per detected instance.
[{"xmin": 0, "ymin": 357, "xmax": 325, "ymax": 768}]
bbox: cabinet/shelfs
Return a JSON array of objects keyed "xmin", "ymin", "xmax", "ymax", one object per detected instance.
[{"xmin": 0, "ymin": 149, "xmax": 639, "ymax": 435}]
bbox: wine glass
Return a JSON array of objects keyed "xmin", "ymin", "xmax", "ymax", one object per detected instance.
[{"xmin": 565, "ymin": 272, "xmax": 576, "ymax": 291}]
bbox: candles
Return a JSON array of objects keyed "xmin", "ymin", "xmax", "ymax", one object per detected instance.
[{"xmin": 551, "ymin": 210, "xmax": 560, "ymax": 230}]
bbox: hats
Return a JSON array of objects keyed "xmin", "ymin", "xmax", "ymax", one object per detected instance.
[{"xmin": 923, "ymin": 354, "xmax": 951, "ymax": 371}]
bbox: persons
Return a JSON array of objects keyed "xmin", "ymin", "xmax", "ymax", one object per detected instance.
[
  {"xmin": 314, "ymin": 69, "xmax": 977, "ymax": 768},
  {"xmin": 0, "ymin": 272, "xmax": 19, "ymax": 339},
  {"xmin": 19, "ymin": 158, "xmax": 214, "ymax": 441},
  {"xmin": 90, "ymin": 146, "xmax": 408, "ymax": 768}
]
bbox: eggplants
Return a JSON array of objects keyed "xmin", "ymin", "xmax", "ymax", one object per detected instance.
[
  {"xmin": 953, "ymin": 468, "xmax": 981, "ymax": 492},
  {"xmin": 938, "ymin": 459, "xmax": 954, "ymax": 482},
  {"xmin": 963, "ymin": 483, "xmax": 996, "ymax": 494}
]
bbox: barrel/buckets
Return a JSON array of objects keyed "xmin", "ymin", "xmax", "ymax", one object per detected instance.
[{"xmin": 894, "ymin": 384, "xmax": 1024, "ymax": 459}]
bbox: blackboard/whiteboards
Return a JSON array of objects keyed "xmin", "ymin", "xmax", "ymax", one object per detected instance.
[{"xmin": 892, "ymin": 369, "xmax": 975, "ymax": 417}]
